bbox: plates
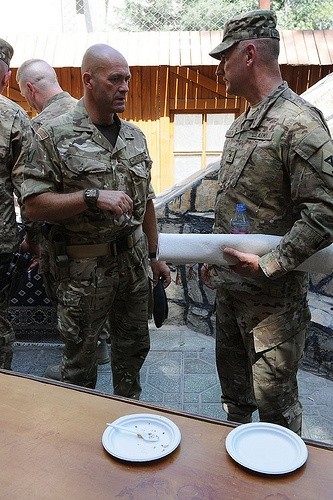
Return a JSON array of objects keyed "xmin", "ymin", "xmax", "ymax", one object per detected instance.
[
  {"xmin": 225, "ymin": 422, "xmax": 308, "ymax": 475},
  {"xmin": 101, "ymin": 413, "xmax": 181, "ymax": 463}
]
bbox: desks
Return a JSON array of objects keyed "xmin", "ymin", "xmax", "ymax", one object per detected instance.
[{"xmin": 0, "ymin": 369, "xmax": 333, "ymax": 500}]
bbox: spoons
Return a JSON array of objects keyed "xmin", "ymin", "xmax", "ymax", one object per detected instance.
[{"xmin": 106, "ymin": 422, "xmax": 160, "ymax": 442}]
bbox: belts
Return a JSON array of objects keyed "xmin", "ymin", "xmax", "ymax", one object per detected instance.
[{"xmin": 65, "ymin": 223, "xmax": 143, "ymax": 257}]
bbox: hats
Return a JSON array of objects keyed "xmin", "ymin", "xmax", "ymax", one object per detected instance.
[
  {"xmin": 208, "ymin": 10, "xmax": 280, "ymax": 60},
  {"xmin": 0, "ymin": 38, "xmax": 14, "ymax": 67}
]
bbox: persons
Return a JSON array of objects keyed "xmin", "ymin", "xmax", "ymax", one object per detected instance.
[
  {"xmin": 0, "ymin": 37, "xmax": 171, "ymax": 401},
  {"xmin": 200, "ymin": 10, "xmax": 333, "ymax": 437}
]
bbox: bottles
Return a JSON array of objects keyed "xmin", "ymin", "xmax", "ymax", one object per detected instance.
[{"xmin": 229, "ymin": 203, "xmax": 252, "ymax": 277}]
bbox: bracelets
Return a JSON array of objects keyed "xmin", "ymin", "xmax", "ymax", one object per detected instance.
[{"xmin": 149, "ymin": 252, "xmax": 160, "ymax": 260}]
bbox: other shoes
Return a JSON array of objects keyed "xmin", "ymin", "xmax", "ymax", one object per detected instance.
[
  {"xmin": 45, "ymin": 366, "xmax": 61, "ymax": 380},
  {"xmin": 96, "ymin": 340, "xmax": 110, "ymax": 364}
]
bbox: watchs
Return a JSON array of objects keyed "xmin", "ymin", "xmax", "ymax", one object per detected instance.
[{"xmin": 83, "ymin": 187, "xmax": 100, "ymax": 210}]
